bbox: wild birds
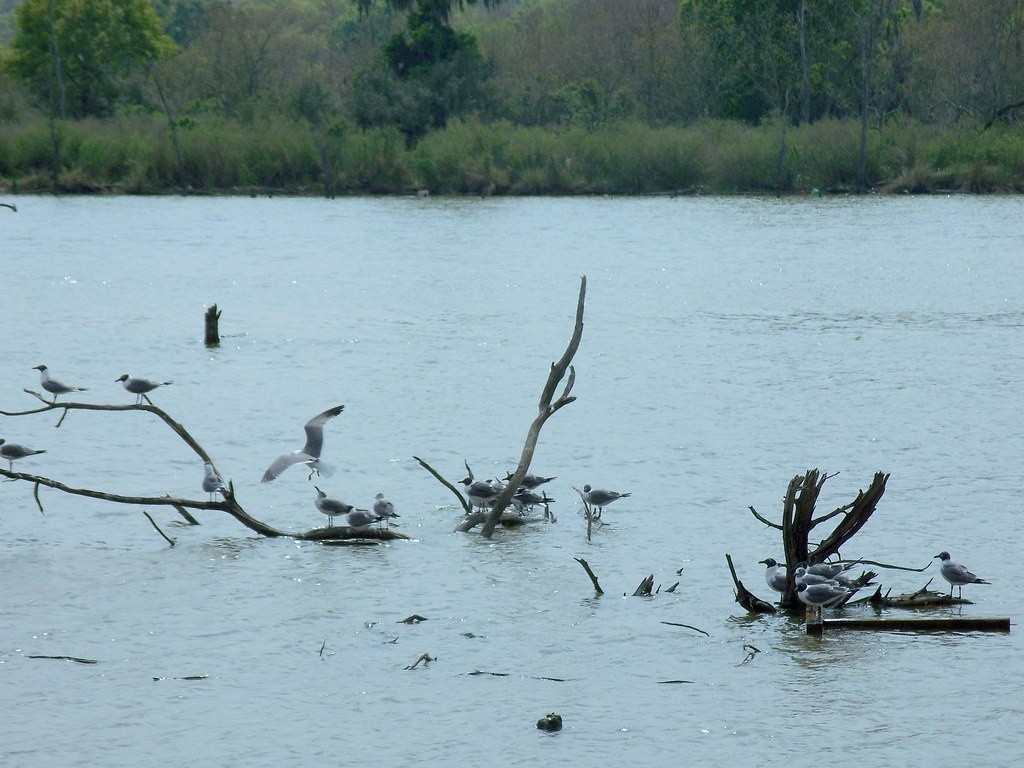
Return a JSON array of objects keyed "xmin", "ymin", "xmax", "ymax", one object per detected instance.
[
  {"xmin": 114, "ymin": 374, "xmax": 174, "ymax": 405},
  {"xmin": 314, "ymin": 485, "xmax": 401, "ymax": 530},
  {"xmin": 202, "ymin": 460, "xmax": 225, "ymax": 504},
  {"xmin": 458, "ymin": 472, "xmax": 556, "ymax": 518},
  {"xmin": 260, "ymin": 404, "xmax": 345, "ymax": 483},
  {"xmin": 0, "ymin": 438, "xmax": 47, "ymax": 474},
  {"xmin": 583, "ymin": 484, "xmax": 631, "ymax": 518},
  {"xmin": 934, "ymin": 551, "xmax": 992, "ymax": 600},
  {"xmin": 758, "ymin": 558, "xmax": 878, "ymax": 623},
  {"xmin": 32, "ymin": 364, "xmax": 90, "ymax": 404}
]
